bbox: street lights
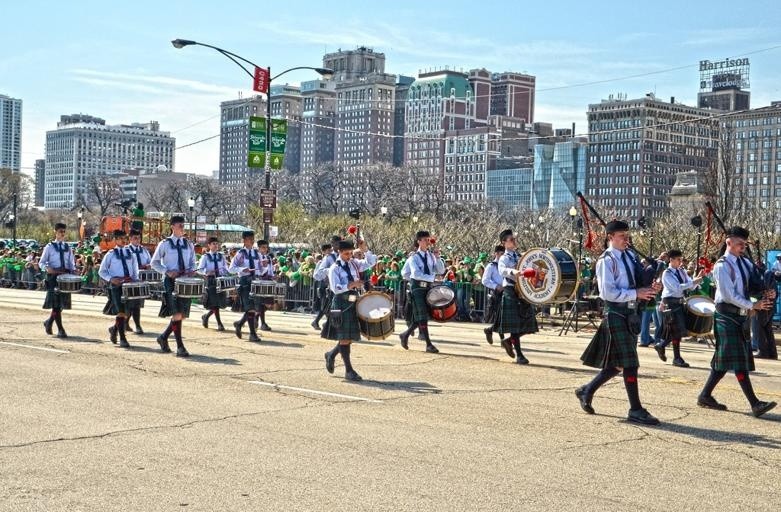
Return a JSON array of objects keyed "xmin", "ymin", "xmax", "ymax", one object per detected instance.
[
  {"xmin": 188, "ymin": 195, "xmax": 195, "ymax": 242},
  {"xmin": 569, "ymin": 205, "xmax": 577, "ymax": 222},
  {"xmin": 170, "ymin": 39, "xmax": 336, "ymax": 244},
  {"xmin": 77, "ymin": 206, "xmax": 87, "ymax": 235},
  {"xmin": 380, "ymin": 204, "xmax": 387, "ymax": 219},
  {"xmin": 12, "ymin": 193, "xmax": 17, "ymax": 246}
]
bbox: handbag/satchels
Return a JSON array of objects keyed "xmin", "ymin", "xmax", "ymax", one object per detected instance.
[
  {"xmin": 329, "ymin": 309, "xmax": 342, "ymax": 327},
  {"xmin": 628, "ymin": 316, "xmax": 641, "ymax": 333},
  {"xmin": 519, "ymin": 302, "xmax": 532, "ymax": 317},
  {"xmin": 741, "ymin": 322, "xmax": 750, "ymax": 341}
]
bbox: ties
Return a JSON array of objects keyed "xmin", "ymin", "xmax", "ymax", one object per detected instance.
[
  {"xmin": 177, "ymin": 239, "xmax": 184, "ymax": 269},
  {"xmin": 622, "ymin": 252, "xmax": 635, "ymax": 288},
  {"xmin": 249, "ymin": 250, "xmax": 255, "ymax": 274},
  {"xmin": 345, "ymin": 262, "xmax": 353, "ymax": 281},
  {"xmin": 120, "ymin": 248, "xmax": 141, "ymax": 277},
  {"xmin": 424, "ymin": 253, "xmax": 428, "ymax": 274},
  {"xmin": 59, "ymin": 243, "xmax": 64, "ymax": 268},
  {"xmin": 676, "ymin": 270, "xmax": 683, "ymax": 283},
  {"xmin": 736, "ymin": 257, "xmax": 747, "ymax": 297},
  {"xmin": 214, "ymin": 254, "xmax": 219, "ymax": 277}
]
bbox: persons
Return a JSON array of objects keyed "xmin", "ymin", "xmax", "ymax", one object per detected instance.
[
  {"xmin": 0, "ymin": 246, "xmax": 49, "ymax": 291},
  {"xmin": 38, "ymin": 216, "xmax": 279, "ymax": 357},
  {"xmin": 312, "ymin": 228, "xmax": 544, "ymax": 382},
  {"xmin": 276, "ymin": 248, "xmax": 322, "ymax": 315},
  {"xmin": 576, "ymin": 218, "xmax": 781, "ymax": 422}
]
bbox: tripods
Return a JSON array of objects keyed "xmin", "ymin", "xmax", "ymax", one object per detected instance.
[{"xmin": 557, "ymin": 238, "xmax": 598, "ymax": 336}]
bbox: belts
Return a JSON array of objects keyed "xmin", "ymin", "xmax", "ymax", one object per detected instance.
[
  {"xmin": 414, "ymin": 280, "xmax": 430, "ymax": 287},
  {"xmin": 612, "ymin": 302, "xmax": 636, "ymax": 309},
  {"xmin": 717, "ymin": 304, "xmax": 747, "ymax": 316},
  {"xmin": 337, "ymin": 295, "xmax": 357, "ymax": 302},
  {"xmin": 664, "ymin": 299, "xmax": 684, "ymax": 304}
]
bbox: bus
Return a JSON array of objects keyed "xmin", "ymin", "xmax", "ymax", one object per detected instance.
[{"xmin": 221, "ymin": 241, "xmax": 316, "ymax": 256}]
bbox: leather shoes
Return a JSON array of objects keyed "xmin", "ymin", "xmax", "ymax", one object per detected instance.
[
  {"xmin": 697, "ymin": 395, "xmax": 726, "ymax": 410},
  {"xmin": 202, "ymin": 315, "xmax": 208, "ymax": 327},
  {"xmin": 655, "ymin": 343, "xmax": 689, "ymax": 367},
  {"xmin": 575, "ymin": 386, "xmax": 594, "ymax": 413},
  {"xmin": 628, "ymin": 409, "xmax": 658, "ymax": 425},
  {"xmin": 325, "ymin": 352, "xmax": 361, "ymax": 381},
  {"xmin": 44, "ymin": 320, "xmax": 67, "ymax": 337},
  {"xmin": 399, "ymin": 333, "xmax": 408, "ymax": 349},
  {"xmin": 109, "ymin": 324, "xmax": 142, "ymax": 347},
  {"xmin": 311, "ymin": 320, "xmax": 321, "ymax": 330},
  {"xmin": 157, "ymin": 336, "xmax": 188, "ymax": 356},
  {"xmin": 218, "ymin": 324, "xmax": 224, "ymax": 331},
  {"xmin": 426, "ymin": 345, "xmax": 438, "ymax": 353},
  {"xmin": 234, "ymin": 322, "xmax": 271, "ymax": 342},
  {"xmin": 752, "ymin": 401, "xmax": 776, "ymax": 417},
  {"xmin": 484, "ymin": 328, "xmax": 529, "ymax": 363}
]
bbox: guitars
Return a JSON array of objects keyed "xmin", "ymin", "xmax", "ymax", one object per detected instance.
[{"xmin": 685, "ymin": 295, "xmax": 717, "ymax": 337}]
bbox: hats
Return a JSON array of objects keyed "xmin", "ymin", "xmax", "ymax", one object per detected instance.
[
  {"xmin": 726, "ymin": 226, "xmax": 749, "ymax": 240},
  {"xmin": 495, "ymin": 229, "xmax": 512, "ymax": 251},
  {"xmin": 257, "ymin": 240, "xmax": 266, "ymax": 245},
  {"xmin": 208, "ymin": 237, "xmax": 217, "ymax": 243},
  {"xmin": 606, "ymin": 220, "xmax": 629, "ymax": 233},
  {"xmin": 416, "ymin": 231, "xmax": 429, "ymax": 238},
  {"xmin": 171, "ymin": 216, "xmax": 184, "ymax": 224},
  {"xmin": 322, "ymin": 242, "xmax": 352, "ymax": 250},
  {"xmin": 55, "ymin": 223, "xmax": 66, "ymax": 228},
  {"xmin": 113, "ymin": 229, "xmax": 140, "ymax": 235},
  {"xmin": 668, "ymin": 250, "xmax": 681, "ymax": 257}
]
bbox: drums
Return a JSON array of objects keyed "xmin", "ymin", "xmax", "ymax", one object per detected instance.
[
  {"xmin": 173, "ymin": 278, "xmax": 204, "ymax": 298},
  {"xmin": 216, "ymin": 276, "xmax": 236, "ymax": 291},
  {"xmin": 275, "ymin": 282, "xmax": 287, "ymax": 297},
  {"xmin": 428, "ymin": 285, "xmax": 458, "ymax": 322},
  {"xmin": 139, "ymin": 268, "xmax": 162, "ymax": 286},
  {"xmin": 357, "ymin": 291, "xmax": 395, "ymax": 340},
  {"xmin": 57, "ymin": 273, "xmax": 82, "ymax": 293},
  {"xmin": 517, "ymin": 248, "xmax": 581, "ymax": 304},
  {"xmin": 251, "ymin": 280, "xmax": 277, "ymax": 297},
  {"xmin": 121, "ymin": 282, "xmax": 151, "ymax": 300}
]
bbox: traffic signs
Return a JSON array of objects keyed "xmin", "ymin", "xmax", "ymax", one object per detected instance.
[{"xmin": 258, "ymin": 190, "xmax": 277, "ymax": 208}]
